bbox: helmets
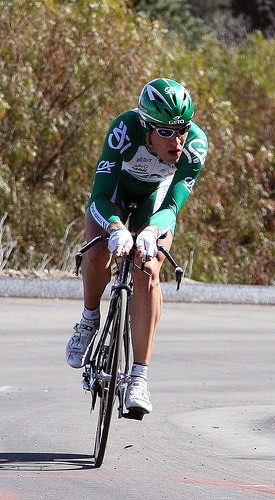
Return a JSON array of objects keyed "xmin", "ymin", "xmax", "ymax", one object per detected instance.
[{"xmin": 139, "ymin": 77, "xmax": 194, "ymax": 133}]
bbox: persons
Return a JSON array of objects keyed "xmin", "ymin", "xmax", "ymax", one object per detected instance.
[{"xmin": 66, "ymin": 77, "xmax": 209, "ymax": 414}]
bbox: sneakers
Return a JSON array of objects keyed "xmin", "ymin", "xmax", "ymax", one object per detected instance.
[
  {"xmin": 66, "ymin": 315, "xmax": 100, "ymax": 370},
  {"xmin": 125, "ymin": 380, "xmax": 153, "ymax": 414}
]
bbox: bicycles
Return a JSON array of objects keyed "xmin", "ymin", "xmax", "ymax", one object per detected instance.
[{"xmin": 75, "ymin": 195, "xmax": 183, "ymax": 468}]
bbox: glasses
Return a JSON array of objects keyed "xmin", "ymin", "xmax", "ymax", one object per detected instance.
[{"xmin": 148, "ymin": 123, "xmax": 192, "ymax": 138}]
